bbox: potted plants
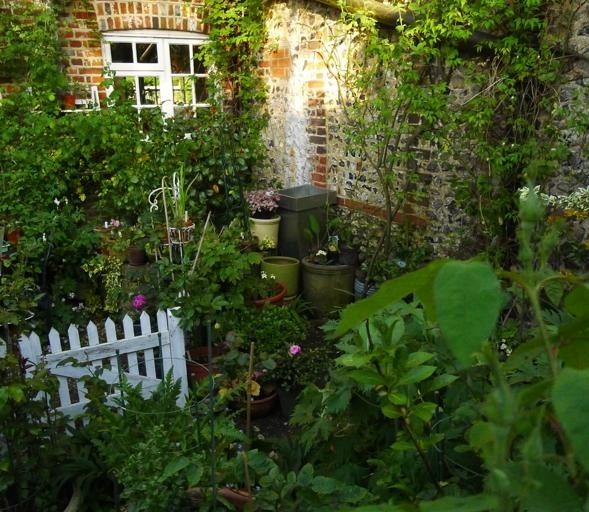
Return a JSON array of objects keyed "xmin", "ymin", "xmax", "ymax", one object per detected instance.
[
  {"xmin": 163, "ymin": 163, "xmax": 196, "ymax": 264},
  {"xmin": 301, "ymin": 215, "xmax": 366, "ymax": 317},
  {"xmin": 329, "ymin": 12, "xmax": 506, "ymax": 303},
  {"xmin": 114, "ymin": 224, "xmax": 165, "ymax": 266},
  {"xmin": 248, "ymin": 270, "xmax": 287, "ymax": 311}
]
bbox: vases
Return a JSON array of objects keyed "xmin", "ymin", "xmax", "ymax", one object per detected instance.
[
  {"xmin": 232, "ymin": 385, "xmax": 278, "ymax": 421},
  {"xmin": 248, "ymin": 214, "xmax": 281, "ymax": 250}
]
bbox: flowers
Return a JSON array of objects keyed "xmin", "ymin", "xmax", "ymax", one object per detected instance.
[
  {"xmin": 213, "ymin": 336, "xmax": 282, "ymax": 399},
  {"xmin": 246, "ymin": 187, "xmax": 281, "ymax": 218}
]
